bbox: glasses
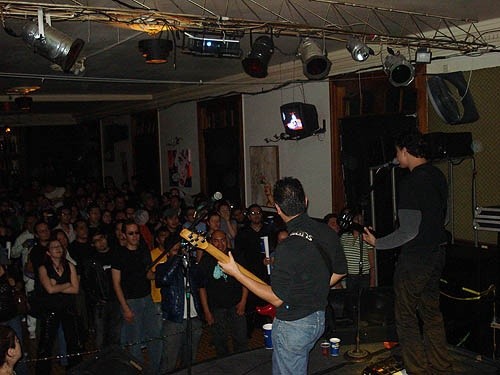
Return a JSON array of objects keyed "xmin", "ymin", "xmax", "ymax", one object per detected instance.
[
  {"xmin": 126, "ymin": 231, "xmax": 139, "ymax": 235},
  {"xmin": 248, "ymin": 211, "xmax": 262, "ymax": 215}
]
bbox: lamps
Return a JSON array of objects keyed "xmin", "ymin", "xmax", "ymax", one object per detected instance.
[
  {"xmin": 344, "ymin": 39, "xmax": 372, "ymax": 60},
  {"xmin": 18, "ymin": 20, "xmax": 85, "ymax": 73},
  {"xmin": 414, "ymin": 47, "xmax": 432, "ymax": 63},
  {"xmin": 138, "ymin": 38, "xmax": 174, "ymax": 64},
  {"xmin": 184, "ymin": 30, "xmax": 242, "ymax": 59},
  {"xmin": 298, "ymin": 37, "xmax": 334, "ymax": 79},
  {"xmin": 383, "ymin": 55, "xmax": 417, "ymax": 88},
  {"xmin": 6, "ymin": 86, "xmax": 40, "ymax": 112},
  {"xmin": 241, "ymin": 33, "xmax": 283, "ymax": 78}
]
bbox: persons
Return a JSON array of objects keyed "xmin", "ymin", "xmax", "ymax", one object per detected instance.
[
  {"xmin": 364, "ymin": 131, "xmax": 454, "ymax": 375},
  {"xmin": 0, "ymin": 176, "xmax": 377, "ymax": 375}
]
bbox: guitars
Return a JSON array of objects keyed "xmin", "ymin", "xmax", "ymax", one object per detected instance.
[
  {"xmin": 336, "ymin": 215, "xmax": 453, "ymax": 273},
  {"xmin": 179, "ymin": 228, "xmax": 279, "ymax": 320}
]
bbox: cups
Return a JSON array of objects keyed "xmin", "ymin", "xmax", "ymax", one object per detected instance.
[
  {"xmin": 262, "ymin": 323, "xmax": 273, "ymax": 349},
  {"xmin": 330, "ymin": 337, "xmax": 341, "ymax": 357},
  {"xmin": 320, "ymin": 342, "xmax": 331, "ymax": 357}
]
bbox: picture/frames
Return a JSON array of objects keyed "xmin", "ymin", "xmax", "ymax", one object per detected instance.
[{"xmin": 246, "ymin": 145, "xmax": 279, "ymax": 208}]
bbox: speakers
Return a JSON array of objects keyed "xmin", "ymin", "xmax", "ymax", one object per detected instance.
[{"xmin": 62, "ymin": 346, "xmax": 158, "ymax": 375}]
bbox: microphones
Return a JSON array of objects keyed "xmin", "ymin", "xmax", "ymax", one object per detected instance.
[
  {"xmin": 198, "ymin": 192, "xmax": 222, "ymax": 210},
  {"xmin": 380, "ymin": 157, "xmax": 400, "ymax": 167}
]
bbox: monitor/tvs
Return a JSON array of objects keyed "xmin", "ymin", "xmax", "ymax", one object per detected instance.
[{"xmin": 280, "ymin": 102, "xmax": 318, "ymax": 136}]
